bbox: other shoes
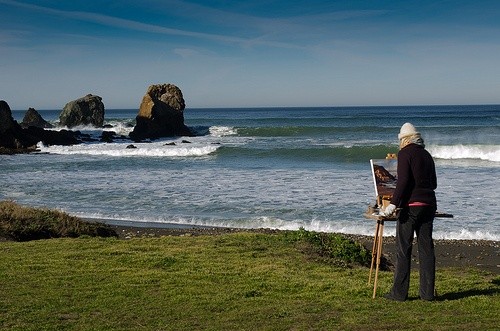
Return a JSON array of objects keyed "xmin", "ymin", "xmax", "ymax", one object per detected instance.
[
  {"xmin": 420, "ymin": 295, "xmax": 433, "ymax": 301},
  {"xmin": 384, "ymin": 293, "xmax": 404, "ymax": 301}
]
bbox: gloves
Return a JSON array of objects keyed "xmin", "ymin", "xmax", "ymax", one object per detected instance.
[{"xmin": 384, "ymin": 203, "xmax": 396, "ymax": 217}]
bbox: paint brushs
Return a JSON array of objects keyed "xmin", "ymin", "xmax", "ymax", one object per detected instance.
[{"xmin": 372, "ymin": 207, "xmax": 404, "ymax": 211}]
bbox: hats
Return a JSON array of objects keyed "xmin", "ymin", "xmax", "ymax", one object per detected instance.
[{"xmin": 398, "ymin": 122, "xmax": 421, "ymax": 140}]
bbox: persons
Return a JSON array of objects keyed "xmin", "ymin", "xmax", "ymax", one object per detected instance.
[{"xmin": 384, "ymin": 122, "xmax": 438, "ymax": 302}]
